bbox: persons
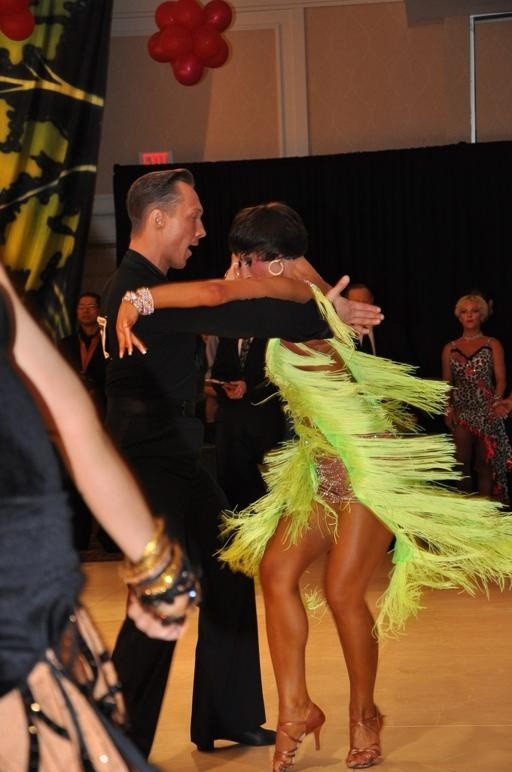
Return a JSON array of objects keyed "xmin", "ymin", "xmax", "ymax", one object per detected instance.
[
  {"xmin": 59, "ymin": 293, "xmax": 118, "ymax": 555},
  {"xmin": 437, "ymin": 293, "xmax": 512, "ymax": 516},
  {"xmin": 1, "ymin": 261, "xmax": 201, "ymax": 772},
  {"xmin": 90, "ymin": 161, "xmax": 387, "ymax": 760},
  {"xmin": 491, "ymin": 396, "xmax": 511, "ymax": 418},
  {"xmin": 345, "ymin": 282, "xmax": 388, "ymax": 358},
  {"xmin": 210, "ymin": 335, "xmax": 269, "ymax": 497},
  {"xmin": 94, "ymin": 190, "xmax": 510, "ymax": 770}
]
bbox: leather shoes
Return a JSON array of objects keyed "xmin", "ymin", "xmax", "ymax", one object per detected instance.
[{"xmin": 197, "ymin": 727, "xmax": 276, "ymax": 752}]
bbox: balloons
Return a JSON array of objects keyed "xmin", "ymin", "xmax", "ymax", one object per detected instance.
[
  {"xmin": 1, "ymin": 7, "xmax": 38, "ymax": 42},
  {"xmin": 147, "ymin": 0, "xmax": 234, "ymax": 86},
  {"xmin": 0, "ymin": 0, "xmax": 29, "ymax": 16}
]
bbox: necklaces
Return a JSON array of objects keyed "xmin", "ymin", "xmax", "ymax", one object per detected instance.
[{"xmin": 461, "ymin": 334, "xmax": 484, "ymax": 343}]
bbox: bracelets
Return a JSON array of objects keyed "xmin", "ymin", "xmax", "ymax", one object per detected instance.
[
  {"xmin": 114, "ymin": 524, "xmax": 198, "ymax": 626},
  {"xmin": 492, "ymin": 393, "xmax": 504, "ymax": 400}
]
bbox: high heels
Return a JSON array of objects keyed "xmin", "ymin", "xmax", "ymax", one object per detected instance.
[
  {"xmin": 346, "ymin": 705, "xmax": 383, "ymax": 769},
  {"xmin": 273, "ymin": 704, "xmax": 325, "ymax": 771}
]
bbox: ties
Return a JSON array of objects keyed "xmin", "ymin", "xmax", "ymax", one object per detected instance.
[{"xmin": 239, "ymin": 337, "xmax": 250, "ymax": 371}]
